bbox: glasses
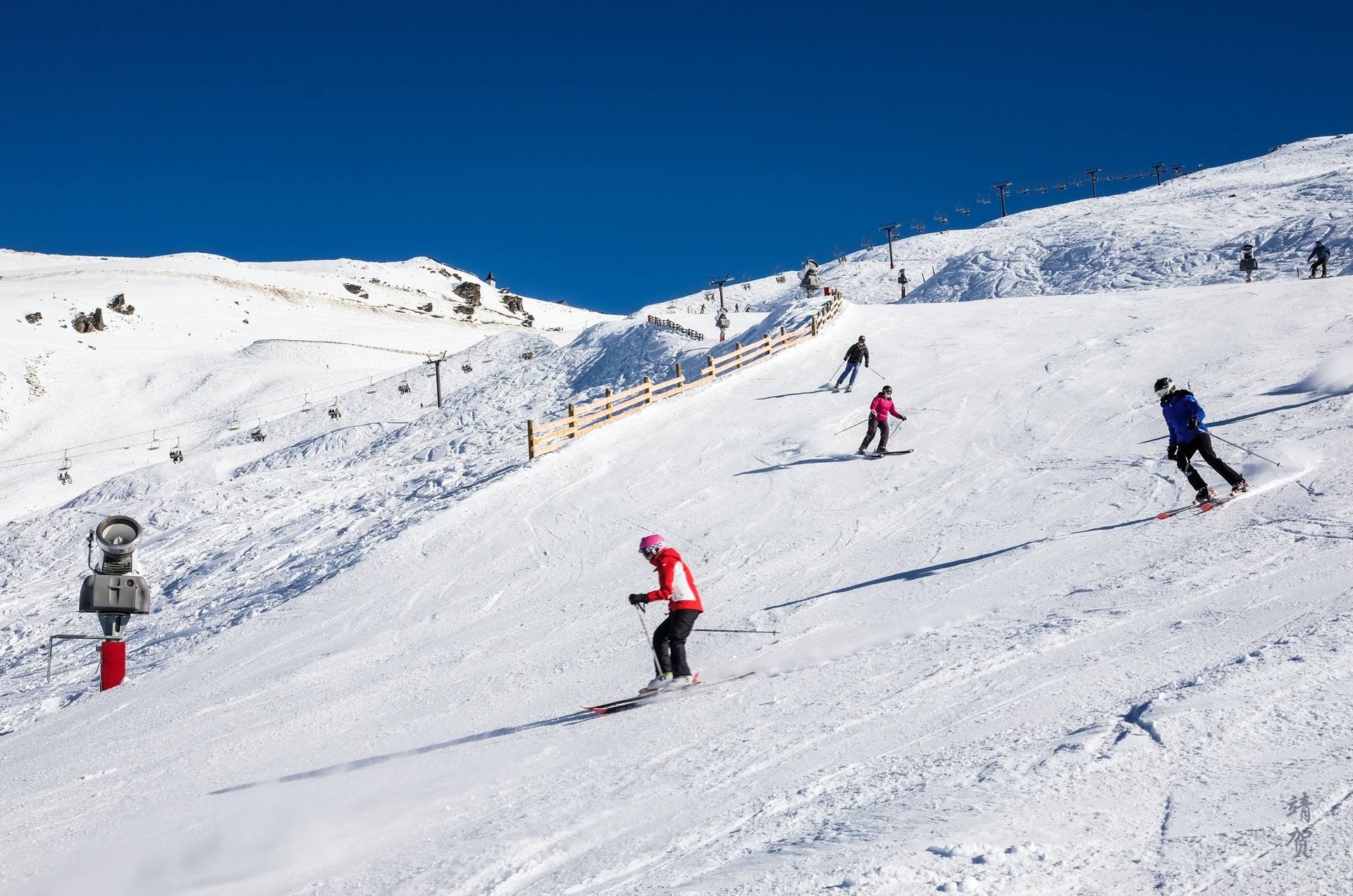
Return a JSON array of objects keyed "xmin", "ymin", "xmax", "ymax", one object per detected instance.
[
  {"xmin": 884, "ymin": 389, "xmax": 892, "ymax": 394},
  {"xmin": 642, "ymin": 541, "xmax": 663, "ymax": 557}
]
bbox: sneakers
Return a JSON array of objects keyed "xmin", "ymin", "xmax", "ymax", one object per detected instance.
[
  {"xmin": 665, "ymin": 675, "xmax": 693, "ymax": 690},
  {"xmin": 1309, "ymin": 273, "xmax": 1316, "ymax": 277},
  {"xmin": 848, "ymin": 384, "xmax": 854, "ymax": 392},
  {"xmin": 835, "ymin": 384, "xmax": 841, "ymax": 390},
  {"xmin": 1322, "ymin": 272, "xmax": 1326, "ymax": 277},
  {"xmin": 876, "ymin": 447, "xmax": 889, "ymax": 452},
  {"xmin": 1192, "ymin": 485, "xmax": 1218, "ymax": 504},
  {"xmin": 859, "ymin": 446, "xmax": 867, "ymax": 454},
  {"xmin": 1230, "ymin": 478, "xmax": 1250, "ymax": 496},
  {"xmin": 648, "ymin": 671, "xmax": 674, "ymax": 689}
]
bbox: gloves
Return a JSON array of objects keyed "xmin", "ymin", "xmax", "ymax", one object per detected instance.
[
  {"xmin": 1308, "ymin": 257, "xmax": 1311, "ymax": 262},
  {"xmin": 843, "ymin": 354, "xmax": 849, "ymax": 361},
  {"xmin": 899, "ymin": 413, "xmax": 906, "ymax": 421},
  {"xmin": 629, "ymin": 593, "xmax": 649, "ymax": 604},
  {"xmin": 1167, "ymin": 446, "xmax": 1177, "ymax": 461},
  {"xmin": 865, "ymin": 362, "xmax": 869, "ymax": 367},
  {"xmin": 1186, "ymin": 418, "xmax": 1199, "ymax": 431},
  {"xmin": 871, "ymin": 410, "xmax": 878, "ymax": 417}
]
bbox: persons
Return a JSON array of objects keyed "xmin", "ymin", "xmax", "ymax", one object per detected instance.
[
  {"xmin": 835, "ymin": 335, "xmax": 869, "ymax": 390},
  {"xmin": 776, "ymin": 276, "xmax": 786, "ymax": 283},
  {"xmin": 629, "ymin": 534, "xmax": 704, "ymax": 692},
  {"xmin": 1003, "ymin": 167, "xmax": 1167, "ymax": 197},
  {"xmin": 1154, "ymin": 378, "xmax": 1246, "ymax": 501},
  {"xmin": 838, "ymin": 256, "xmax": 847, "ymax": 263},
  {"xmin": 744, "ymin": 284, "xmax": 750, "ymax": 290},
  {"xmin": 523, "ymin": 352, "xmax": 534, "ymax": 360},
  {"xmin": 706, "ymin": 294, "xmax": 715, "ymax": 301},
  {"xmin": 60, "ymin": 471, "xmax": 73, "ymax": 485},
  {"xmin": 892, "ymin": 207, "xmax": 970, "ymax": 240},
  {"xmin": 700, "ymin": 304, "xmax": 706, "ymax": 314},
  {"xmin": 252, "ymin": 430, "xmax": 265, "ymax": 442},
  {"xmin": 1307, "ymin": 241, "xmax": 1330, "ymax": 278},
  {"xmin": 859, "ymin": 385, "xmax": 906, "ymax": 453},
  {"xmin": 735, "ymin": 303, "xmax": 739, "ymax": 313},
  {"xmin": 399, "ymin": 384, "xmax": 411, "ymax": 394},
  {"xmin": 866, "ymin": 245, "xmax": 872, "ymax": 252},
  {"xmin": 329, "ymin": 408, "xmax": 342, "ymax": 419},
  {"xmin": 462, "ymin": 364, "xmax": 473, "ymax": 373},
  {"xmin": 170, "ymin": 450, "xmax": 183, "ymax": 464}
]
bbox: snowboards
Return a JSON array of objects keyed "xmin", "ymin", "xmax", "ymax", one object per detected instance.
[{"xmin": 1301, "ymin": 275, "xmax": 1334, "ymax": 280}]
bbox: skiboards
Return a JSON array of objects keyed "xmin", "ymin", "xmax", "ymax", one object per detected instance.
[
  {"xmin": 1157, "ymin": 485, "xmax": 1255, "ymax": 519},
  {"xmin": 580, "ymin": 672, "xmax": 702, "ymax": 715},
  {"xmin": 855, "ymin": 448, "xmax": 914, "ymax": 458},
  {"xmin": 832, "ymin": 390, "xmax": 852, "ymax": 393}
]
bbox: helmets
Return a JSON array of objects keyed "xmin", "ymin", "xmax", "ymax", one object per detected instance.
[
  {"xmin": 1315, "ymin": 241, "xmax": 1323, "ymax": 247},
  {"xmin": 858, "ymin": 335, "xmax": 866, "ymax": 341},
  {"xmin": 1154, "ymin": 377, "xmax": 1176, "ymax": 399},
  {"xmin": 882, "ymin": 386, "xmax": 892, "ymax": 392},
  {"xmin": 638, "ymin": 534, "xmax": 665, "ymax": 553}
]
